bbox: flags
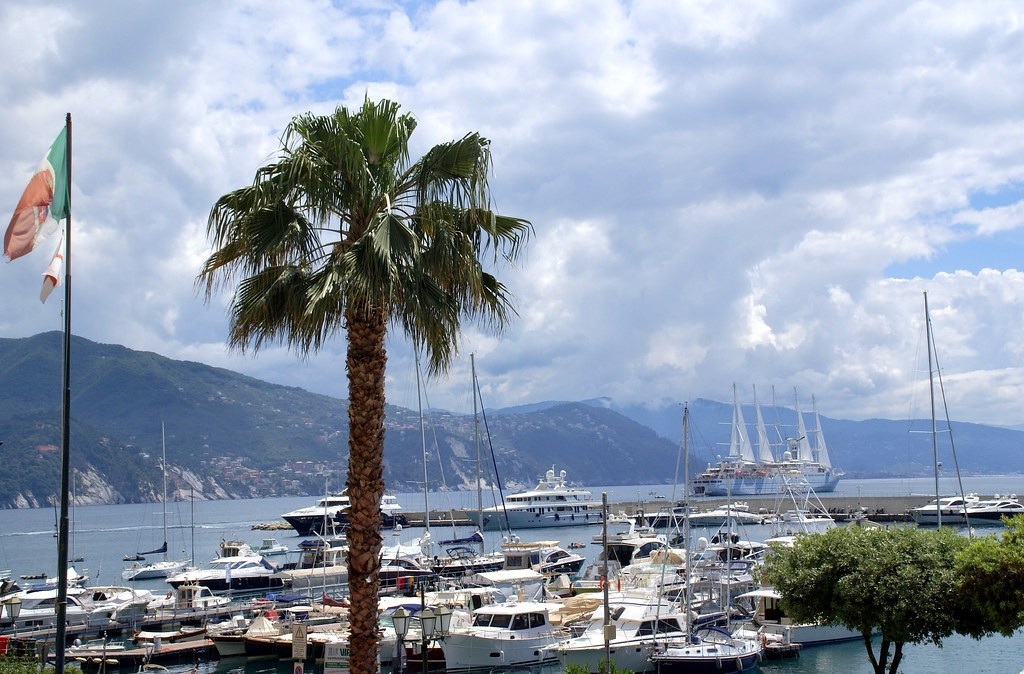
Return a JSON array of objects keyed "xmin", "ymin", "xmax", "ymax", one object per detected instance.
[
  {"xmin": 4, "ymin": 124, "xmax": 70, "ymax": 261},
  {"xmin": 40, "ymin": 238, "xmax": 64, "ymax": 303}
]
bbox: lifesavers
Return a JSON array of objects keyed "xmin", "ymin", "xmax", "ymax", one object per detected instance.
[
  {"xmin": 600, "ymin": 577, "xmax": 610, "ymax": 590},
  {"xmin": 618, "ymin": 579, "xmax": 620, "ymax": 593},
  {"xmin": 762, "ymin": 635, "xmax": 767, "ymax": 644}
]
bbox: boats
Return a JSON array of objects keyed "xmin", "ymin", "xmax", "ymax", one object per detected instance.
[
  {"xmin": 460, "ymin": 464, "xmax": 610, "ymax": 531},
  {"xmin": 904, "ymin": 493, "xmax": 980, "ymax": 526},
  {"xmin": 0, "ymin": 290, "xmax": 980, "ymax": 674},
  {"xmin": 959, "ymin": 492, "xmax": 1024, "ymax": 526},
  {"xmin": 279, "ymin": 486, "xmax": 410, "ymax": 536}
]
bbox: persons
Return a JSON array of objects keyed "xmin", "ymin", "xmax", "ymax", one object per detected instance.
[
  {"xmin": 814, "ymin": 509, "xmax": 817, "ymax": 513},
  {"xmin": 876, "ymin": 509, "xmax": 884, "ymax": 514},
  {"xmin": 828, "ymin": 508, "xmax": 844, "ymax": 513}
]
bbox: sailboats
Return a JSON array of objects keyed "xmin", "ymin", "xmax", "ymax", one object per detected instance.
[{"xmin": 689, "ymin": 382, "xmax": 846, "ymax": 497}]
